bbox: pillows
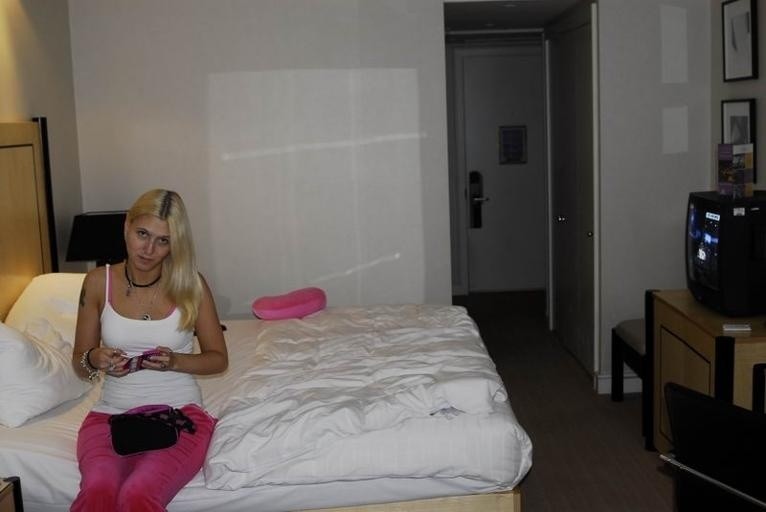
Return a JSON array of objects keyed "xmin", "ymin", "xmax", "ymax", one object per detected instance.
[
  {"xmin": 0, "ymin": 318, "xmax": 93, "ymax": 429},
  {"xmin": 5, "ymin": 271, "xmax": 87, "ymax": 346},
  {"xmin": 251, "ymin": 286, "xmax": 327, "ymax": 320}
]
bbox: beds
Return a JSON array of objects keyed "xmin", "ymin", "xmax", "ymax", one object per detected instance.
[{"xmin": 0, "ymin": 112, "xmax": 537, "ymax": 512}]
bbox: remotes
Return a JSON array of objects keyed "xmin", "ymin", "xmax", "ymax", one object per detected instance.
[{"xmin": 723, "ymin": 324, "xmax": 752, "ymax": 331}]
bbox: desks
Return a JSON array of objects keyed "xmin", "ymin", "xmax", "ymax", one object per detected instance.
[{"xmin": 644, "ymin": 287, "xmax": 765, "ymax": 454}]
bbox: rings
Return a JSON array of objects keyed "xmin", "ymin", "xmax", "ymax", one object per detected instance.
[{"xmin": 161, "ymin": 363, "xmax": 167, "ymax": 370}]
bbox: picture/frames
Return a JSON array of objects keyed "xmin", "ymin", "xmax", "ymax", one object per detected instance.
[
  {"xmin": 722, "ymin": 1, "xmax": 759, "ymax": 83},
  {"xmin": 721, "ymin": 97, "xmax": 758, "ymax": 184}
]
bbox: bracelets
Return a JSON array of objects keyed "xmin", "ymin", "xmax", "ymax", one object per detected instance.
[
  {"xmin": 87, "ymin": 348, "xmax": 97, "ymax": 370},
  {"xmin": 81, "ymin": 348, "xmax": 115, "ymax": 385}
]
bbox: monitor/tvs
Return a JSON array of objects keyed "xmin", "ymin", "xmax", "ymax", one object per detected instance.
[{"xmin": 685, "ymin": 190, "xmax": 766, "ymax": 318}]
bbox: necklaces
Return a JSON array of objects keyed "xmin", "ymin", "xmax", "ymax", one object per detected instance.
[
  {"xmin": 124, "ymin": 262, "xmax": 162, "ymax": 289},
  {"xmin": 131, "ymin": 278, "xmax": 159, "ymax": 321}
]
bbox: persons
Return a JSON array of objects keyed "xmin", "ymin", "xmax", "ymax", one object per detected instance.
[{"xmin": 69, "ymin": 189, "xmax": 229, "ymax": 512}]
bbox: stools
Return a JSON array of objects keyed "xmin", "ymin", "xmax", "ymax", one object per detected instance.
[
  {"xmin": 611, "ymin": 318, "xmax": 645, "ymax": 433},
  {"xmin": 660, "ymin": 365, "xmax": 765, "ymax": 512}
]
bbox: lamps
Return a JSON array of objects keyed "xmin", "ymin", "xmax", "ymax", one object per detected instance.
[{"xmin": 65, "ymin": 209, "xmax": 128, "ymax": 270}]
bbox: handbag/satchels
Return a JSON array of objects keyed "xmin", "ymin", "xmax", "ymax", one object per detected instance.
[{"xmin": 108, "ymin": 405, "xmax": 196, "ymax": 456}]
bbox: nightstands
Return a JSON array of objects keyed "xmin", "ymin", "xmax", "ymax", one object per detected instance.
[{"xmin": 0, "ymin": 476, "xmax": 23, "ymax": 512}]
